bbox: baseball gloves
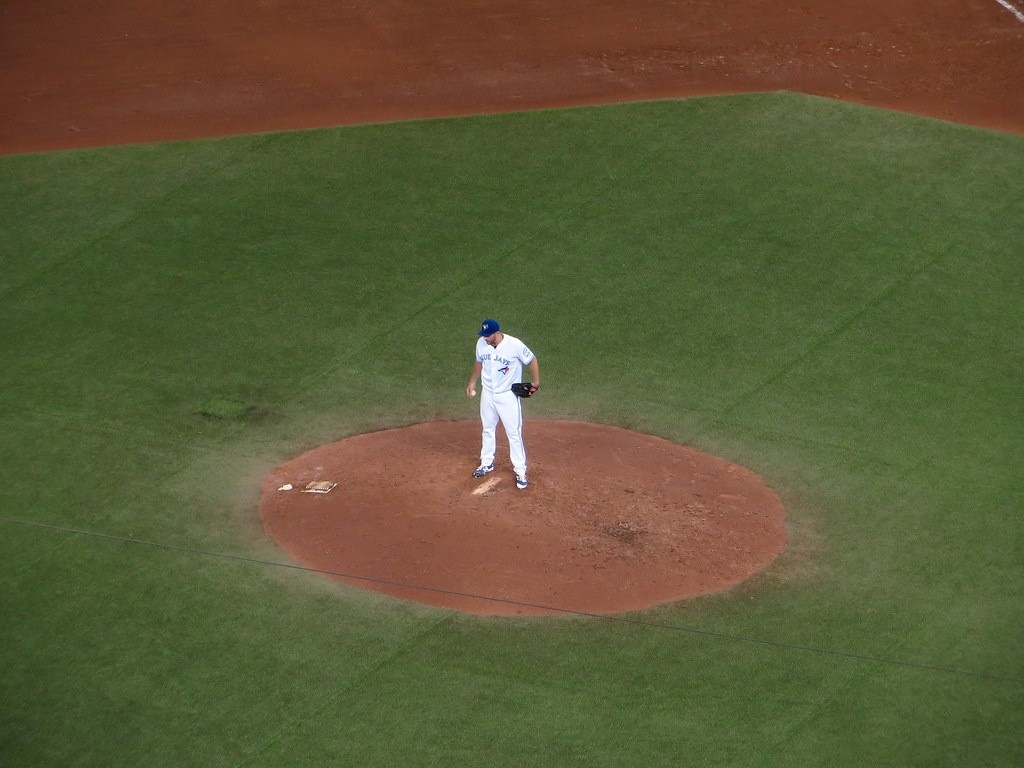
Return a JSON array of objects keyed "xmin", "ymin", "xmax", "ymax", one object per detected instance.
[{"xmin": 511, "ymin": 382, "xmax": 538, "ymax": 398}]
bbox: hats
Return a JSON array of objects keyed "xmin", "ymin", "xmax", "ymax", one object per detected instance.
[{"xmin": 478, "ymin": 319, "xmax": 499, "ymax": 336}]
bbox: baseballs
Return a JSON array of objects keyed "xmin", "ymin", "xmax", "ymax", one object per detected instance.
[{"xmin": 470, "ymin": 390, "xmax": 477, "ymax": 397}]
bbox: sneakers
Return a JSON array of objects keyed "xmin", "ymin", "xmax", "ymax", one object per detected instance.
[
  {"xmin": 516, "ymin": 475, "xmax": 527, "ymax": 488},
  {"xmin": 472, "ymin": 463, "xmax": 494, "ymax": 477}
]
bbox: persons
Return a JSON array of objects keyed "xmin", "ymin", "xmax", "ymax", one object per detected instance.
[{"xmin": 466, "ymin": 317, "xmax": 540, "ymax": 489}]
